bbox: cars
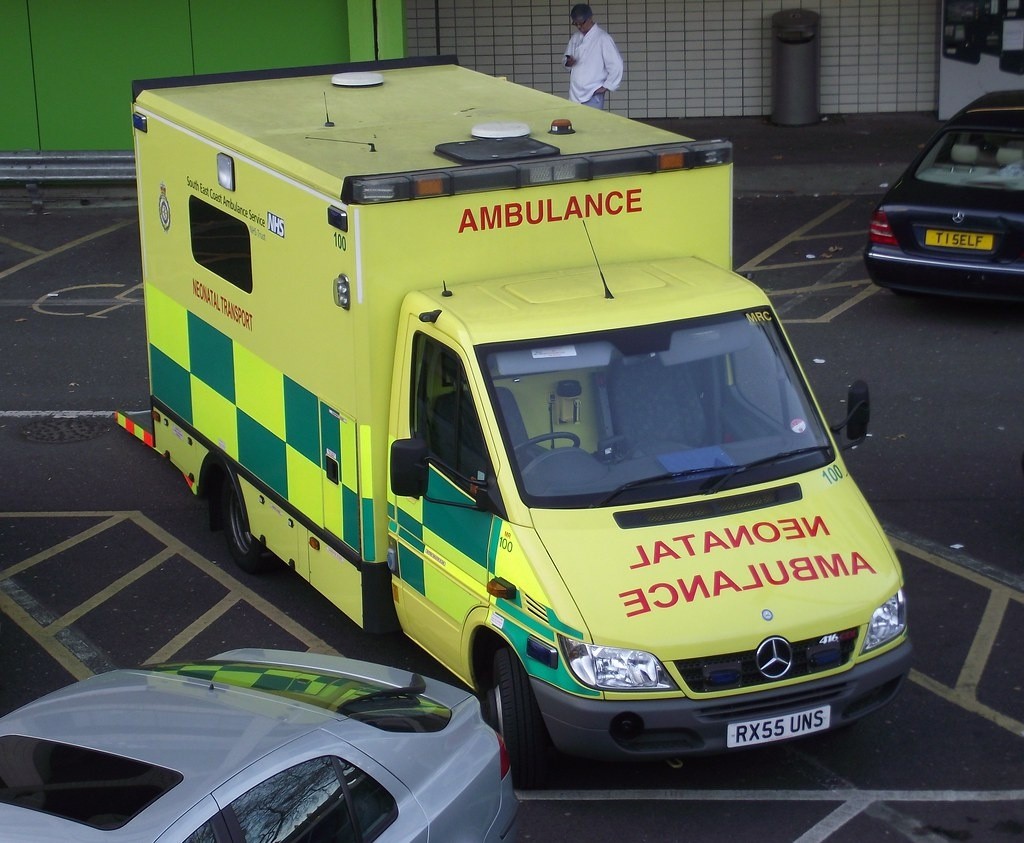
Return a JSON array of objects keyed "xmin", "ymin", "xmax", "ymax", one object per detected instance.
[
  {"xmin": 0, "ymin": 646, "xmax": 521, "ymax": 843},
  {"xmin": 863, "ymin": 90, "xmax": 1023, "ymax": 303}
]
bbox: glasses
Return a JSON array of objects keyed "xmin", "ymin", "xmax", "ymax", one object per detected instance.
[{"xmin": 571, "ymin": 18, "xmax": 588, "ymax": 28}]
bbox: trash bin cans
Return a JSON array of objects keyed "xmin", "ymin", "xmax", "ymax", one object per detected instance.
[{"xmin": 770, "ymin": 8, "xmax": 822, "ymax": 127}]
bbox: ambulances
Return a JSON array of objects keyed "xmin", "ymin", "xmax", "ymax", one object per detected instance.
[{"xmin": 115, "ymin": 53, "xmax": 914, "ymax": 786}]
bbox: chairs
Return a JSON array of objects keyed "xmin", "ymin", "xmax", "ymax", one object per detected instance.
[
  {"xmin": 433, "ymin": 386, "xmax": 553, "ymax": 489},
  {"xmin": 611, "ymin": 346, "xmax": 710, "ymax": 465},
  {"xmin": 996, "ymin": 148, "xmax": 1023, "ymax": 171},
  {"xmin": 949, "ymin": 144, "xmax": 981, "ymax": 176}
]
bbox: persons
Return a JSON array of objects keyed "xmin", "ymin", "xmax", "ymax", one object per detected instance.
[{"xmin": 561, "ymin": 3, "xmax": 624, "ymax": 110}]
